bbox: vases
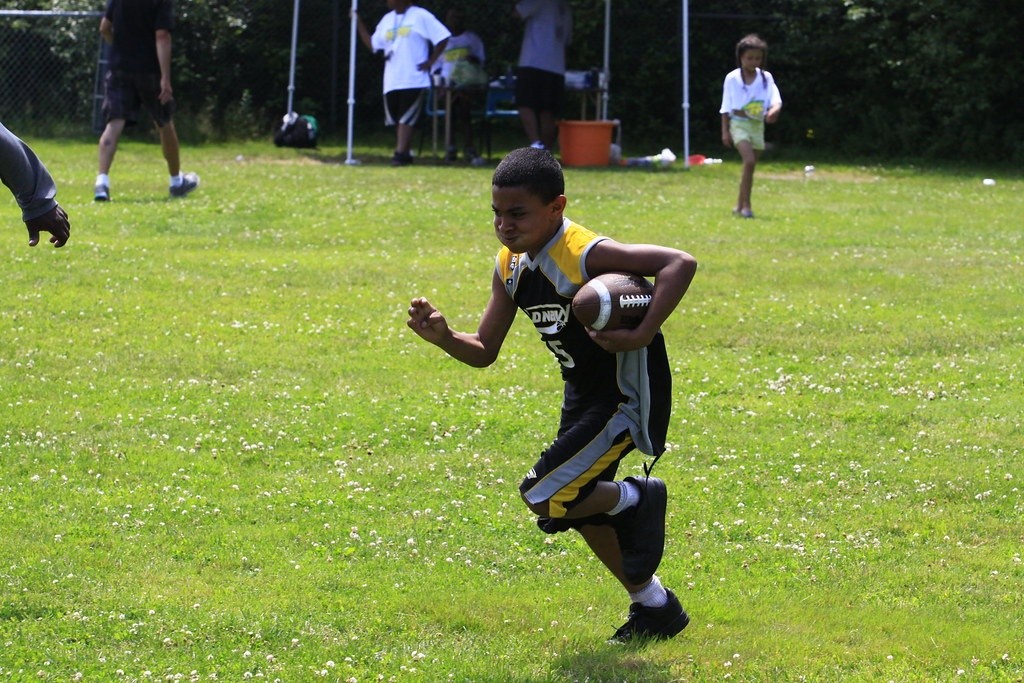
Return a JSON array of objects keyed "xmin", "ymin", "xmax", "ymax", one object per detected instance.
[{"xmin": 557, "ymin": 119, "xmax": 615, "ymax": 167}]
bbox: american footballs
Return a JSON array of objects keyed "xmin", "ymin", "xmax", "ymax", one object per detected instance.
[{"xmin": 573, "ymin": 270, "xmax": 657, "ymax": 331}]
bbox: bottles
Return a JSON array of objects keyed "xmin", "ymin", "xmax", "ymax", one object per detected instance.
[
  {"xmin": 805, "ymin": 165, "xmax": 816, "ymax": 194},
  {"xmin": 307, "ymin": 121, "xmax": 315, "ymax": 139}
]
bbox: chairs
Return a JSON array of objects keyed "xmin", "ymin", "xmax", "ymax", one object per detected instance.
[{"xmin": 416, "ymin": 74, "xmax": 449, "ymax": 160}]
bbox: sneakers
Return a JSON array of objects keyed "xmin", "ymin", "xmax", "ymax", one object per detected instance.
[
  {"xmin": 607, "ymin": 475, "xmax": 668, "ymax": 585},
  {"xmin": 94, "ymin": 183, "xmax": 109, "ymax": 202},
  {"xmin": 169, "ymin": 171, "xmax": 200, "ymax": 199},
  {"xmin": 610, "ymin": 587, "xmax": 690, "ymax": 645}
]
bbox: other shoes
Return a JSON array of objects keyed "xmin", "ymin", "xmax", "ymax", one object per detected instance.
[
  {"xmin": 732, "ymin": 208, "xmax": 742, "ymax": 215},
  {"xmin": 462, "ymin": 145, "xmax": 484, "ymax": 165},
  {"xmin": 390, "ymin": 152, "xmax": 413, "ymax": 167},
  {"xmin": 443, "ymin": 146, "xmax": 460, "ymax": 165},
  {"xmin": 532, "ymin": 140, "xmax": 546, "ymax": 150},
  {"xmin": 740, "ymin": 208, "xmax": 753, "ymax": 218}
]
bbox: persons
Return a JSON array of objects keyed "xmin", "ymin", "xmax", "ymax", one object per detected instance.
[
  {"xmin": 348, "ymin": 0, "xmax": 452, "ymax": 171},
  {"xmin": 425, "ymin": 7, "xmax": 492, "ymax": 166},
  {"xmin": 406, "ymin": 144, "xmax": 698, "ymax": 652},
  {"xmin": 89, "ymin": 1, "xmax": 205, "ymax": 205},
  {"xmin": 499, "ymin": 0, "xmax": 585, "ymax": 161},
  {"xmin": 717, "ymin": 30, "xmax": 785, "ymax": 222},
  {"xmin": 0, "ymin": 116, "xmax": 72, "ymax": 250}
]
